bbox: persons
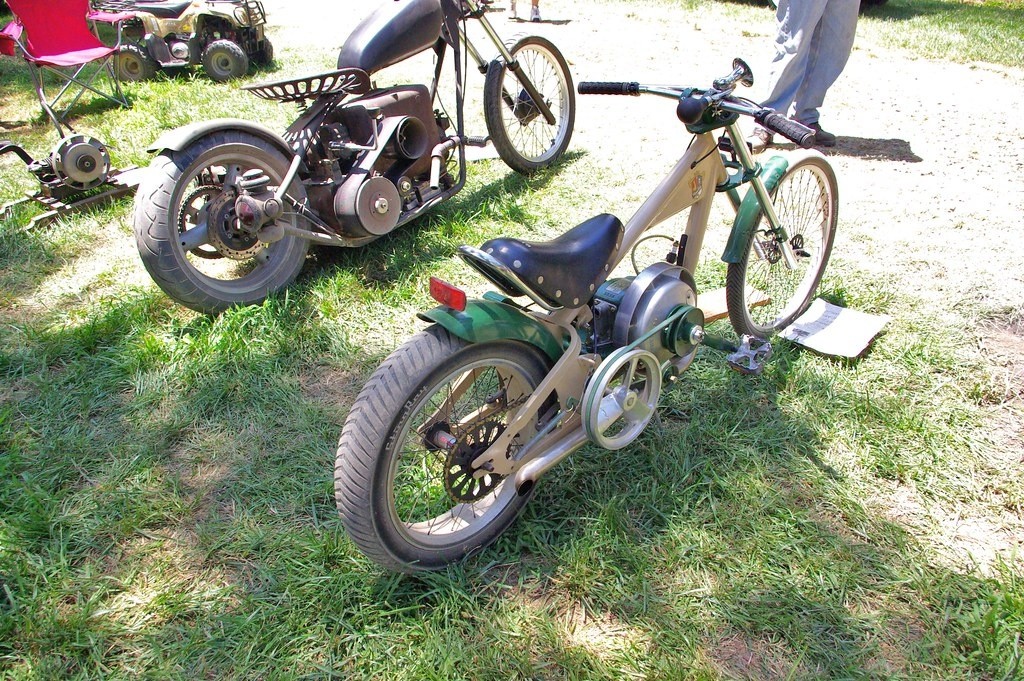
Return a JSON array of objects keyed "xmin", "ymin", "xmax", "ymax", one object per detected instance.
[
  {"xmin": 745, "ymin": 0, "xmax": 861, "ymax": 153},
  {"xmin": 506, "ymin": 0, "xmax": 542, "ymax": 21}
]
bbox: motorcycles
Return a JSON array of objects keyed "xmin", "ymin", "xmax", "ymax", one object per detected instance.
[
  {"xmin": 88, "ymin": 0, "xmax": 274, "ymax": 85},
  {"xmin": 132, "ymin": 0, "xmax": 576, "ymax": 320}
]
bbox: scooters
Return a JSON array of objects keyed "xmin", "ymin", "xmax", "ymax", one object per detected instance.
[{"xmin": 333, "ymin": 56, "xmax": 840, "ymax": 577}]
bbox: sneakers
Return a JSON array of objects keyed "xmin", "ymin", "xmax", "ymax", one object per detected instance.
[
  {"xmin": 806, "ymin": 122, "xmax": 836, "ymax": 146},
  {"xmin": 747, "ymin": 126, "xmax": 773, "ymax": 154}
]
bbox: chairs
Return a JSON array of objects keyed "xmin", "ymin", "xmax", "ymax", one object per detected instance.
[{"xmin": 0, "ymin": 0, "xmax": 137, "ymax": 124}]
